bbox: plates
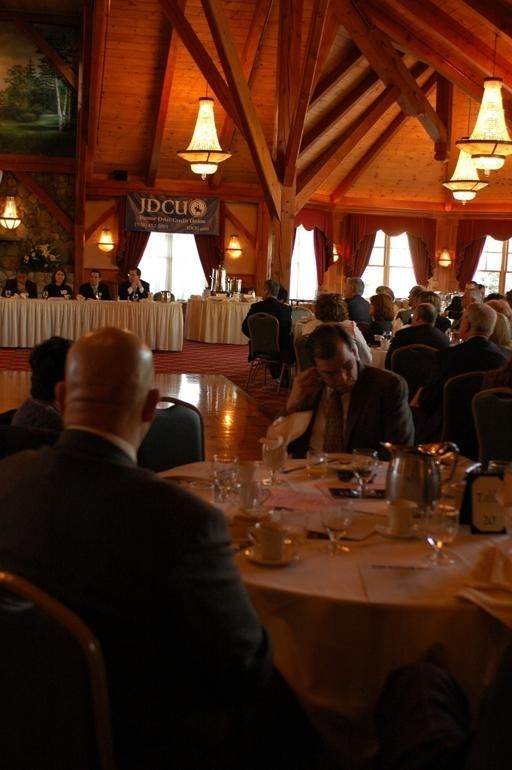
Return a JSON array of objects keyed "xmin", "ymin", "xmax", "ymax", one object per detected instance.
[{"xmin": 243, "ymin": 545, "xmax": 297, "ymax": 567}]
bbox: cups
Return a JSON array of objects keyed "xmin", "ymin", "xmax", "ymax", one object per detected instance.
[
  {"xmin": 488, "ymin": 460, "xmax": 512, "ymax": 474},
  {"xmin": 449, "ymin": 332, "xmax": 460, "ymax": 344},
  {"xmin": 374, "ymin": 335, "xmax": 383, "ymax": 349},
  {"xmin": 382, "ymin": 330, "xmax": 393, "ymax": 340},
  {"xmin": 148, "ymin": 292, "xmax": 154, "ymax": 303},
  {"xmin": 470, "ymin": 455, "xmax": 507, "ymax": 535},
  {"xmin": 212, "ymin": 451, "xmax": 418, "ymax": 559}
]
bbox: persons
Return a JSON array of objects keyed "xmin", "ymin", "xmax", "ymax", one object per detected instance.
[
  {"xmin": 118, "ymin": 266, "xmax": 148, "ymax": 300},
  {"xmin": 0, "ymin": 325, "xmax": 344, "ymax": 770},
  {"xmin": 78, "ymin": 270, "xmax": 111, "ymax": 300},
  {"xmin": 240, "ymin": 273, "xmax": 512, "ymax": 463},
  {"xmin": 1, "ymin": 334, "xmax": 76, "ymax": 460},
  {"xmin": 42, "ymin": 268, "xmax": 73, "ymax": 300},
  {"xmin": 1, "ymin": 266, "xmax": 38, "ymax": 298}
]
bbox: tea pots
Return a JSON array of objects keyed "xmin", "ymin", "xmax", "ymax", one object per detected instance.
[{"xmin": 379, "ymin": 439, "xmax": 460, "ymax": 518}]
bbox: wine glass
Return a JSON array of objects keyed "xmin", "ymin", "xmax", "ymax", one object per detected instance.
[
  {"xmin": 262, "ymin": 442, "xmax": 286, "ymax": 486},
  {"xmin": 425, "ymin": 504, "xmax": 459, "ymax": 567},
  {"xmin": 132, "ymin": 293, "xmax": 139, "ymax": 302},
  {"xmin": 42, "ymin": 291, "xmax": 48, "ymax": 300},
  {"xmin": 95, "ymin": 291, "xmax": 103, "ymax": 300},
  {"xmin": 5, "ymin": 290, "xmax": 11, "ymax": 299}
]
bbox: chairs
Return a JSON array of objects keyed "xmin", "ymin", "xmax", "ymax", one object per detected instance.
[
  {"xmin": 138, "ymin": 398, "xmax": 204, "ymax": 472},
  {"xmin": 0, "ymin": 571, "xmax": 115, "ymax": 770},
  {"xmin": 154, "ymin": 292, "xmax": 175, "ymax": 302}
]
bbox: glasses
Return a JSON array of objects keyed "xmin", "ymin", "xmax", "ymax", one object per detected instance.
[{"xmin": 127, "ymin": 273, "xmax": 135, "ymax": 276}]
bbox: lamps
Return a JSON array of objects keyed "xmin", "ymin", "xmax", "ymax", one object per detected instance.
[
  {"xmin": 176, "ymin": 82, "xmax": 232, "ymax": 181},
  {"xmin": 225, "ymin": 233, "xmax": 244, "ymax": 258},
  {"xmin": 442, "ymin": 73, "xmax": 489, "ymax": 206},
  {"xmin": 98, "ymin": 224, "xmax": 115, "ymax": 252},
  {"xmin": 332, "ymin": 242, "xmax": 339, "ymax": 263},
  {"xmin": 438, "ymin": 248, "xmax": 452, "ymax": 268},
  {"xmin": 455, "ymin": 35, "xmax": 512, "ymax": 176},
  {"xmin": 0, "ymin": 192, "xmax": 22, "ymax": 230}
]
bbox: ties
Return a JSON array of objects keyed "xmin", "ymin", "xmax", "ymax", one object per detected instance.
[{"xmin": 322, "ymin": 389, "xmax": 345, "ymax": 453}]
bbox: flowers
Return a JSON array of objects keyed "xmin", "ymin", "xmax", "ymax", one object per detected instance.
[{"xmin": 23, "ymin": 243, "xmax": 63, "ymax": 269}]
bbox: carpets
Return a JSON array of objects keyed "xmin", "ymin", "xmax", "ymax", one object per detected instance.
[{"xmin": 0, "ymin": 340, "xmax": 290, "ymax": 416}]
